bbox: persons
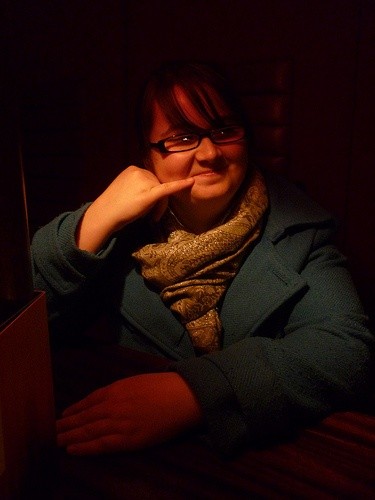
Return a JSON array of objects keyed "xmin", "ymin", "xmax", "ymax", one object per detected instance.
[{"xmin": 29, "ymin": 52, "xmax": 375, "ymax": 500}]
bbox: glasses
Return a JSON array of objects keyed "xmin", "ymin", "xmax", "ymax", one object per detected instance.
[{"xmin": 146, "ymin": 122, "xmax": 246, "ymax": 154}]
